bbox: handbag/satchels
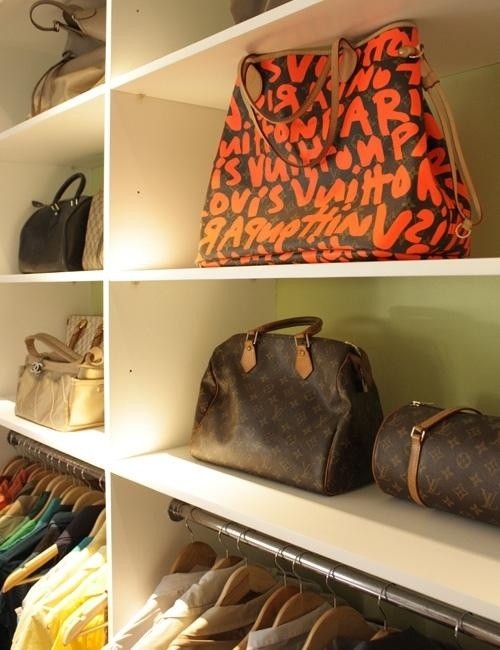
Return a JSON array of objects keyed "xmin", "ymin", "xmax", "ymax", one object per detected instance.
[
  {"xmin": 21, "ymin": 169, "xmax": 85, "ymax": 271},
  {"xmin": 81, "ymin": 186, "xmax": 103, "ymax": 271},
  {"xmin": 15, "ymin": 331, "xmax": 103, "ymax": 431},
  {"xmin": 28, "ymin": 2, "xmax": 106, "ymax": 117},
  {"xmin": 192, "ymin": 315, "xmax": 382, "ymax": 499},
  {"xmin": 66, "ymin": 314, "xmax": 104, "ymax": 357},
  {"xmin": 373, "ymin": 401, "xmax": 499, "ymax": 526},
  {"xmin": 197, "ymin": 20, "xmax": 481, "ymax": 267}
]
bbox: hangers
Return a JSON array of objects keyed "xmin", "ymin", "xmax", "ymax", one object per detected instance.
[{"xmin": 0, "ymin": 427, "xmax": 403, "ymax": 650}]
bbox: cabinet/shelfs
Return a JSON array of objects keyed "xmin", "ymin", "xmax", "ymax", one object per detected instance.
[{"xmin": 0, "ymin": 1, "xmax": 500, "ymax": 650}]
